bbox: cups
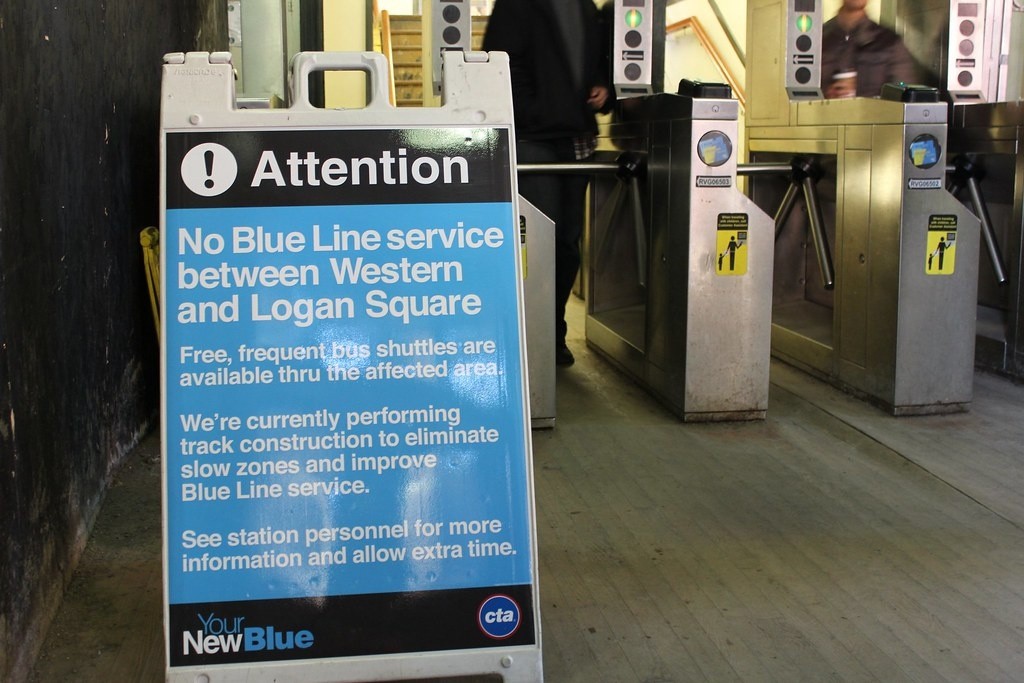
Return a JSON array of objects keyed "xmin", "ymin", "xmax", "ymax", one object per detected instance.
[{"xmin": 834, "ymin": 73, "xmax": 857, "ymax": 98}]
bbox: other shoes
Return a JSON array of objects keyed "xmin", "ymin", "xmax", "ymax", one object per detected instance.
[{"xmin": 555, "ymin": 337, "xmax": 575, "ymax": 368}]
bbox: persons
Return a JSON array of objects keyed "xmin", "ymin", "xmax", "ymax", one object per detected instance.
[
  {"xmin": 480, "ymin": 0, "xmax": 617, "ymax": 366},
  {"xmin": 821, "ymin": 0, "xmax": 918, "ymax": 99}
]
